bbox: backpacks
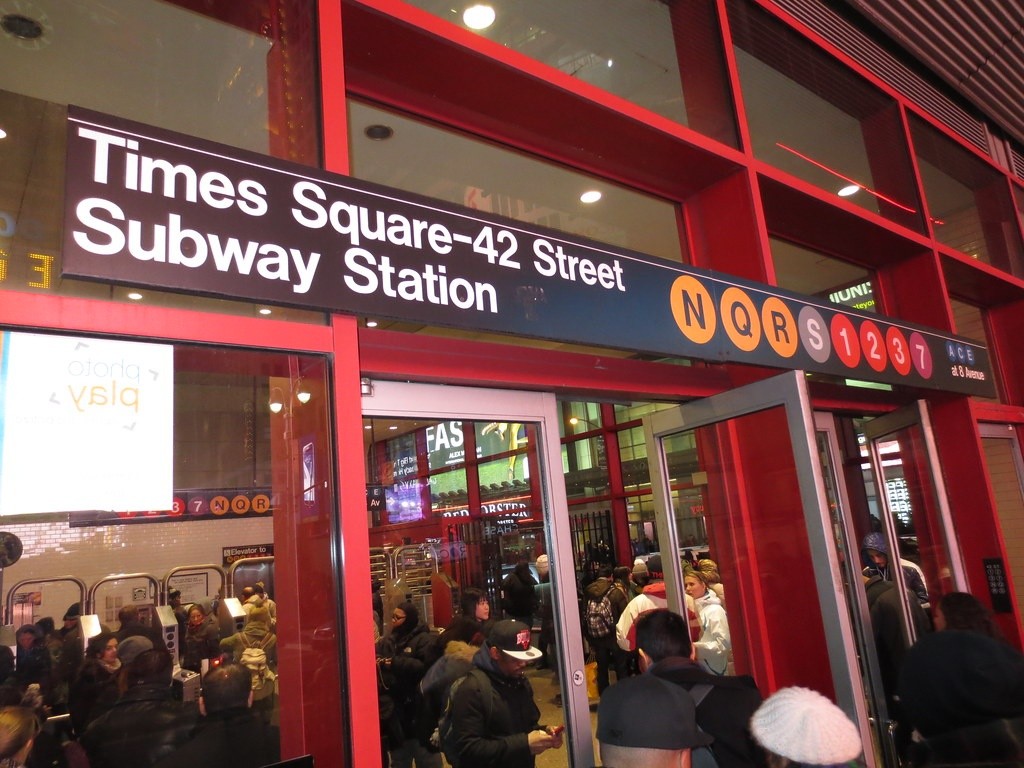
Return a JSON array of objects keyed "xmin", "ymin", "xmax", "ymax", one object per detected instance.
[
  {"xmin": 418, "ymin": 657, "xmax": 493, "ymax": 754},
  {"xmin": 238, "ymin": 629, "xmax": 275, "ymax": 701},
  {"xmin": 585, "ymin": 587, "xmax": 615, "ymax": 638}
]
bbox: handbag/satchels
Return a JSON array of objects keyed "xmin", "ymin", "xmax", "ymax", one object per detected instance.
[{"xmin": 583, "ymin": 662, "xmax": 600, "ymax": 705}]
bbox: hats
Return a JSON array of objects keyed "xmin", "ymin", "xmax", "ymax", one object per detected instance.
[
  {"xmin": 248, "ymin": 598, "xmax": 269, "ymax": 623},
  {"xmin": 62, "ymin": 602, "xmax": 81, "ymax": 621},
  {"xmin": 645, "ymin": 554, "xmax": 664, "ymax": 579},
  {"xmin": 749, "ymin": 686, "xmax": 862, "ymax": 764},
  {"xmin": 170, "ymin": 589, "xmax": 181, "ymax": 600},
  {"xmin": 514, "ymin": 561, "xmax": 533, "ymax": 575},
  {"xmin": 632, "ymin": 559, "xmax": 649, "ymax": 576},
  {"xmin": 489, "ymin": 619, "xmax": 543, "ymax": 660},
  {"xmin": 896, "ymin": 630, "xmax": 1024, "ymax": 740},
  {"xmin": 535, "ymin": 555, "xmax": 549, "ymax": 574},
  {"xmin": 115, "ymin": 636, "xmax": 153, "ymax": 666},
  {"xmin": 596, "ymin": 673, "xmax": 714, "ymax": 749},
  {"xmin": 256, "ymin": 582, "xmax": 264, "ymax": 588}
]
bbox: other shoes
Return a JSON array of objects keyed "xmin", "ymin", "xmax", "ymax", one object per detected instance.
[{"xmin": 536, "ymin": 664, "xmax": 550, "ymax": 670}]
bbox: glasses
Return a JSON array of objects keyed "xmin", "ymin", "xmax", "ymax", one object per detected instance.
[{"xmin": 392, "ymin": 613, "xmax": 407, "ymax": 620}]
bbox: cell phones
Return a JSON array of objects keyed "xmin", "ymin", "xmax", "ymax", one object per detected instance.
[{"xmin": 549, "ymin": 723, "xmax": 566, "ymax": 736}]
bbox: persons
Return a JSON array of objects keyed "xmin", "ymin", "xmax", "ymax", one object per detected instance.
[
  {"xmin": 0, "ymin": 531, "xmax": 1024, "ymax": 768},
  {"xmin": 440, "ymin": 619, "xmax": 563, "ymax": 768}
]
